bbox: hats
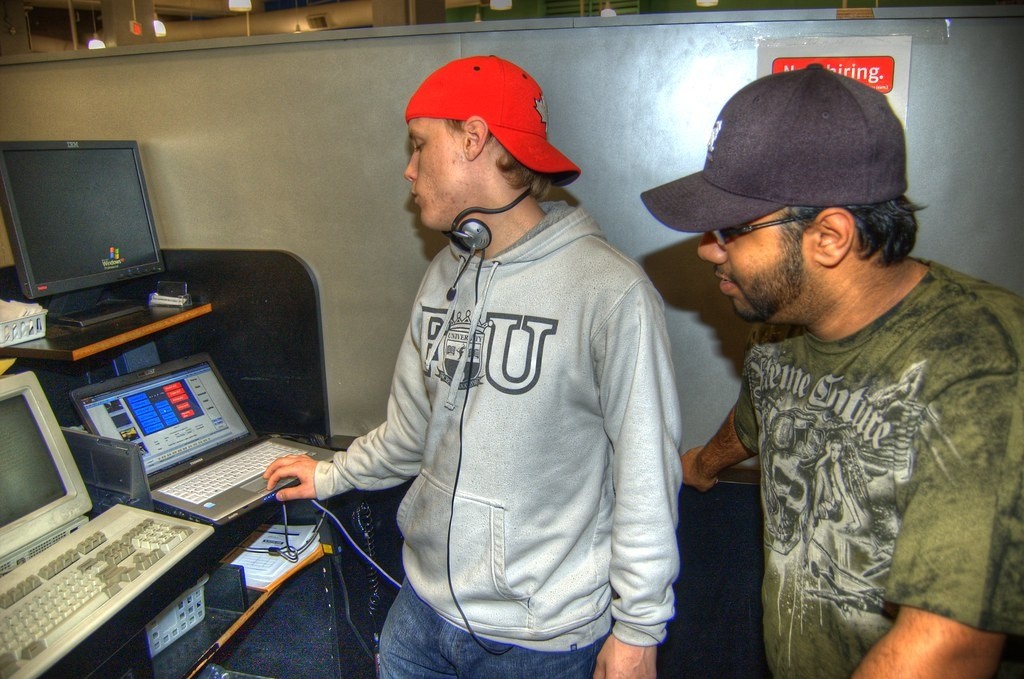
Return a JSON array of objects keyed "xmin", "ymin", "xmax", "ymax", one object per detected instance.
[
  {"xmin": 404, "ymin": 55, "xmax": 581, "ymax": 187},
  {"xmin": 640, "ymin": 62, "xmax": 908, "ymax": 233}
]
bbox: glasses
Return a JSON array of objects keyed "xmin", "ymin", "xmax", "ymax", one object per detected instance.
[{"xmin": 710, "ymin": 210, "xmax": 865, "ymax": 245}]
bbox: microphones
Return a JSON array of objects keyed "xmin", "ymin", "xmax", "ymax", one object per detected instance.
[{"xmin": 446, "ymin": 248, "xmax": 475, "ymax": 301}]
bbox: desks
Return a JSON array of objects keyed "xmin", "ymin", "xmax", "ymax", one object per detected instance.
[{"xmin": 0, "ymin": 247, "xmax": 356, "ymax": 679}]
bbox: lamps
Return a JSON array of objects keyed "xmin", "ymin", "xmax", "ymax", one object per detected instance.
[
  {"xmin": 88, "ymin": 10, "xmax": 106, "ymax": 49},
  {"xmin": 152, "ymin": 7, "xmax": 166, "ymax": 37}
]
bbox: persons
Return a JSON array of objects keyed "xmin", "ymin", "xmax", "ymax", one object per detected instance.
[
  {"xmin": 639, "ymin": 62, "xmax": 1024, "ymax": 679},
  {"xmin": 262, "ymin": 53, "xmax": 684, "ymax": 679}
]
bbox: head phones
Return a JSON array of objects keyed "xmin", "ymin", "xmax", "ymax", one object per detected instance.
[{"xmin": 442, "ymin": 187, "xmax": 533, "ymax": 252}]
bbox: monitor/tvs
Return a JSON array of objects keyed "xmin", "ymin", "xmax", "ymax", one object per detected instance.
[
  {"xmin": 0, "ymin": 139, "xmax": 165, "ymax": 328},
  {"xmin": 0, "ymin": 373, "xmax": 91, "ymax": 577}
]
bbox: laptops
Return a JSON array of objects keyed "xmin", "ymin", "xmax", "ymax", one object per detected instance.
[{"xmin": 69, "ymin": 352, "xmax": 340, "ymax": 527}]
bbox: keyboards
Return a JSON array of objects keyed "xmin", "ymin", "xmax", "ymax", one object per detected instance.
[{"xmin": 0, "ymin": 502, "xmax": 216, "ymax": 679}]
